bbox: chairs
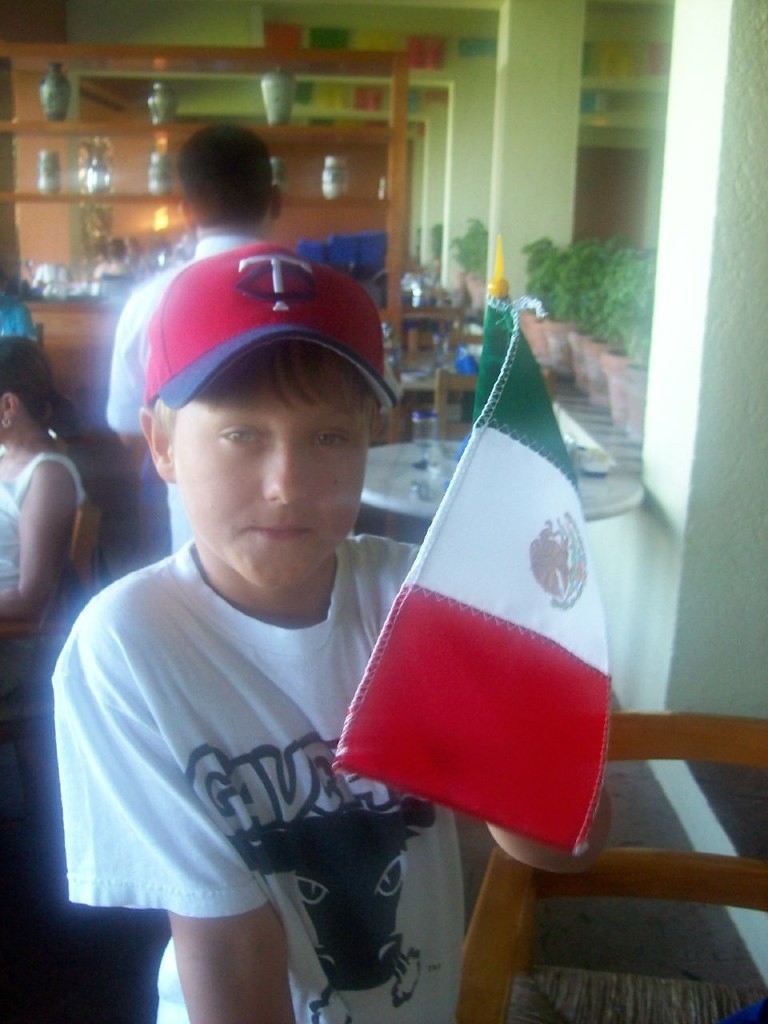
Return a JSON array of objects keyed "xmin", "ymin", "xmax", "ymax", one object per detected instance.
[
  {"xmin": 0, "ymin": 490, "xmax": 100, "ymax": 726},
  {"xmin": 432, "ymin": 358, "xmax": 554, "ymax": 440},
  {"xmin": 457, "ymin": 713, "xmax": 768, "ymax": 1024}
]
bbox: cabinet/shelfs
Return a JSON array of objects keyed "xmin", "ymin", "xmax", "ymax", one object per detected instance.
[{"xmin": 0, "ymin": 41, "xmax": 412, "ymax": 383}]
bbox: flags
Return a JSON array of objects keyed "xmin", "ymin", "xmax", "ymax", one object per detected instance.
[{"xmin": 332, "ymin": 299, "xmax": 610, "ymax": 853}]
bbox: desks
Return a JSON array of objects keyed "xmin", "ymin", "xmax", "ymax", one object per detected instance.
[
  {"xmin": 375, "ymin": 353, "xmax": 476, "ymax": 449},
  {"xmin": 361, "ymin": 439, "xmax": 645, "ymax": 525}
]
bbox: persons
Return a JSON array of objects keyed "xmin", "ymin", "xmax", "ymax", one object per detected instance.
[{"xmin": 0, "ymin": 125, "xmax": 593, "ymax": 1024}]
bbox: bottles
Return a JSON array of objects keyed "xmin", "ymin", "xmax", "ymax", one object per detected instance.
[{"xmin": 412, "ymin": 409, "xmax": 438, "ymax": 476}]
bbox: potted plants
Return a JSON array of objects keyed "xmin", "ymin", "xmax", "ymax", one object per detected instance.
[{"xmin": 429, "ymin": 219, "xmax": 655, "ymax": 441}]
